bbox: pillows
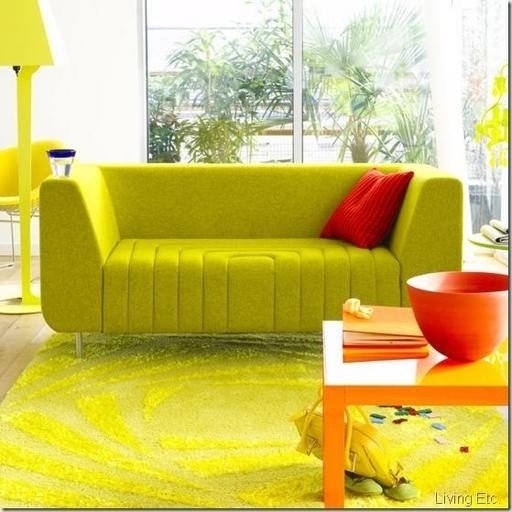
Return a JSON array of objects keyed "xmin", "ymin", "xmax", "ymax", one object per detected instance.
[{"xmin": 321, "ymin": 168, "xmax": 414, "ymax": 248}]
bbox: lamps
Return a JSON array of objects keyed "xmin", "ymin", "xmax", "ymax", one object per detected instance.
[{"xmin": 0, "ymin": 1, "xmax": 56, "ymax": 317}]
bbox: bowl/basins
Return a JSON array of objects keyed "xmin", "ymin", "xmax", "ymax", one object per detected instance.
[{"xmin": 405, "ymin": 270, "xmax": 509, "ymax": 363}]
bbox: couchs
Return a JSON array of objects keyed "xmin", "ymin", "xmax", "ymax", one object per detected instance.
[{"xmin": 34, "ymin": 161, "xmax": 464, "ymax": 356}]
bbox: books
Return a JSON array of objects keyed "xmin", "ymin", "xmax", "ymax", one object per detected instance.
[
  {"xmin": 343, "ymin": 303, "xmax": 428, "ymax": 362},
  {"xmin": 479, "ymin": 218, "xmax": 509, "ymax": 244}
]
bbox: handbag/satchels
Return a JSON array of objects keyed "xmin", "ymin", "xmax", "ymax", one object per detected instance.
[{"xmin": 295, "ymin": 387, "xmax": 404, "ymax": 488}]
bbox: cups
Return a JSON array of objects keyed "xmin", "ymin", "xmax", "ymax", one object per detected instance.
[{"xmin": 45, "ymin": 149, "xmax": 76, "ymax": 178}]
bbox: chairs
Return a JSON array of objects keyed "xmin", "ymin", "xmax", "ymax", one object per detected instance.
[{"xmin": 1, "ymin": 142, "xmax": 65, "ymax": 274}]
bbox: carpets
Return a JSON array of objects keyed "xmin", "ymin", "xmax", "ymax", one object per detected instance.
[{"xmin": 0, "ymin": 335, "xmax": 507, "ymax": 506}]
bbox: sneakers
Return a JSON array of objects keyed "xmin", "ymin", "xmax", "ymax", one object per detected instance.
[{"xmin": 345, "ymin": 470, "xmax": 416, "ymax": 501}]
